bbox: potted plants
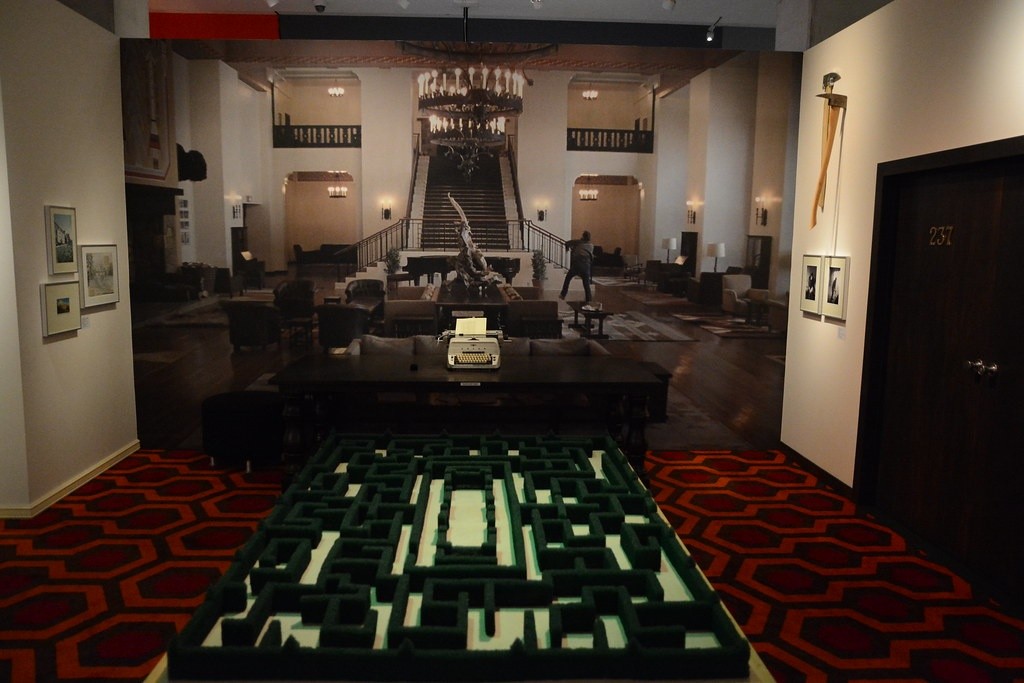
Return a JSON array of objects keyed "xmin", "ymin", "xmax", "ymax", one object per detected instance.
[
  {"xmin": 530, "ymin": 249, "xmax": 548, "ymax": 288},
  {"xmin": 383, "ymin": 248, "xmax": 401, "ymax": 295}
]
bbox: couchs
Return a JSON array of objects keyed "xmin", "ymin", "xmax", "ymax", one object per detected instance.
[{"xmin": 154, "ymin": 246, "xmax": 790, "ymax": 477}]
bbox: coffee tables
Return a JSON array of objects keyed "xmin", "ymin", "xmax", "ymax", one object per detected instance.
[{"xmin": 435, "ymin": 281, "xmax": 507, "ymax": 331}]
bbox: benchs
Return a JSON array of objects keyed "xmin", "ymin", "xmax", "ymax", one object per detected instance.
[{"xmin": 387, "ymin": 273, "xmax": 414, "ymax": 286}]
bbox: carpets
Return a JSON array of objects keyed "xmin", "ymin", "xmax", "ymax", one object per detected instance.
[
  {"xmin": 145, "ymin": 432, "xmax": 777, "ymax": 683},
  {"xmin": 589, "ymin": 275, "xmax": 651, "ymax": 287},
  {"xmin": 620, "ymin": 289, "xmax": 783, "ymax": 339},
  {"xmin": 164, "ymin": 287, "xmax": 277, "ymax": 328},
  {"xmin": 556, "ymin": 310, "xmax": 700, "ymax": 342}
]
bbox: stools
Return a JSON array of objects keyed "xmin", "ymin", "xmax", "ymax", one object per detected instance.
[
  {"xmin": 640, "ymin": 361, "xmax": 673, "ymax": 414},
  {"xmin": 201, "ymin": 389, "xmax": 280, "ymax": 474}
]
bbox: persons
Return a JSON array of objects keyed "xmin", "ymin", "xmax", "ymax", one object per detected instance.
[{"xmin": 558, "ymin": 231, "xmax": 593, "ymax": 302}]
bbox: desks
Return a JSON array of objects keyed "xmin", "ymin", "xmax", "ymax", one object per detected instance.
[
  {"xmin": 324, "ymin": 296, "xmax": 341, "ymax": 304},
  {"xmin": 265, "ymin": 350, "xmax": 665, "ymax": 463},
  {"xmin": 288, "ymin": 317, "xmax": 314, "ymax": 350},
  {"xmin": 567, "ymin": 300, "xmax": 613, "ymax": 339},
  {"xmin": 520, "ymin": 315, "xmax": 564, "ymax": 341},
  {"xmin": 393, "ymin": 313, "xmax": 434, "ymax": 336}
]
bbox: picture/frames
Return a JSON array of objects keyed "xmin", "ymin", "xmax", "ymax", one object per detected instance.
[
  {"xmin": 39, "ymin": 205, "xmax": 118, "ymax": 339},
  {"xmin": 799, "ymin": 254, "xmax": 851, "ymax": 321}
]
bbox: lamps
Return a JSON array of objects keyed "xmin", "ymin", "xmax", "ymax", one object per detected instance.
[
  {"xmin": 230, "ymin": 0, "xmax": 769, "ymax": 226},
  {"xmin": 707, "ymin": 243, "xmax": 725, "ymax": 273},
  {"xmin": 662, "ymin": 237, "xmax": 677, "ymax": 263}
]
